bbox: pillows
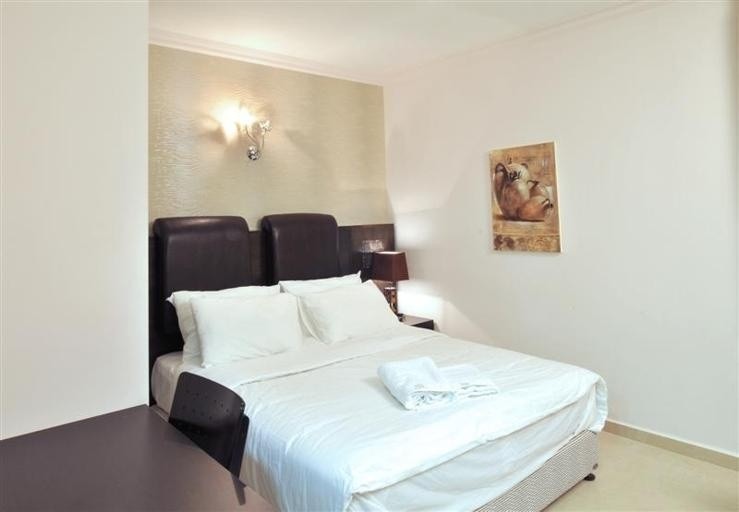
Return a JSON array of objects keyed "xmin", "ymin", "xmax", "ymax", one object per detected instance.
[
  {"xmin": 277, "ymin": 269, "xmax": 363, "ymax": 338},
  {"xmin": 294, "ymin": 278, "xmax": 400, "ymax": 348},
  {"xmin": 188, "ymin": 292, "xmax": 305, "ymax": 368},
  {"xmin": 165, "ymin": 281, "xmax": 282, "ymax": 362}
]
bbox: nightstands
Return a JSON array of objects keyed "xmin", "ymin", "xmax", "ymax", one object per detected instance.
[{"xmin": 397, "ymin": 313, "xmax": 435, "ymax": 332}]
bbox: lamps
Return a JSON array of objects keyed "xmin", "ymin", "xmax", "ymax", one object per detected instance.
[
  {"xmin": 369, "ymin": 250, "xmax": 409, "ymax": 321},
  {"xmin": 233, "ymin": 103, "xmax": 270, "ymax": 160}
]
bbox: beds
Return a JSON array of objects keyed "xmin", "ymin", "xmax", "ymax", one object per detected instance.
[{"xmin": 149, "ymin": 209, "xmax": 610, "ymax": 511}]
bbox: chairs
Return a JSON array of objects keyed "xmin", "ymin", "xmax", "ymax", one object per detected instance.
[{"xmin": 169, "ymin": 371, "xmax": 250, "ymax": 478}]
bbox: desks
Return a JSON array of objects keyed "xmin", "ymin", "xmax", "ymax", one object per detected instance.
[{"xmin": 0, "ymin": 403, "xmax": 284, "ymax": 512}]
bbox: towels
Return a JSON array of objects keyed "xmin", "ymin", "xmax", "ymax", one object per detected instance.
[
  {"xmin": 377, "ymin": 354, "xmax": 459, "ymax": 414},
  {"xmin": 443, "ymin": 362, "xmax": 498, "ymax": 398}
]
